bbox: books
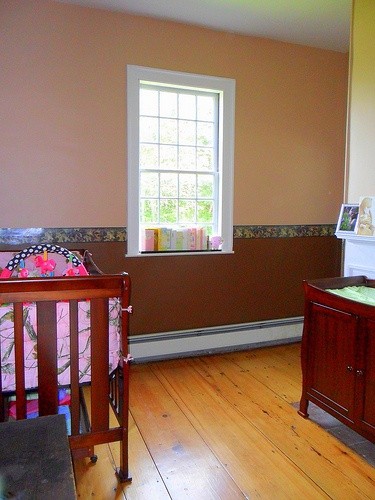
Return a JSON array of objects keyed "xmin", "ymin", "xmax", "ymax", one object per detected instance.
[
  {"xmin": 358, "ymin": 196, "xmax": 375, "ymax": 235},
  {"xmin": 145, "ymin": 227, "xmax": 210, "ymax": 251},
  {"xmin": 336, "ymin": 204, "xmax": 360, "ymax": 234}
]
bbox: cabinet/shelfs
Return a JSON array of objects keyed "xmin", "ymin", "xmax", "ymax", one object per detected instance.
[
  {"xmin": 334, "ymin": 233, "xmax": 375, "ymax": 280},
  {"xmin": 298, "ymin": 275, "xmax": 375, "ymax": 445}
]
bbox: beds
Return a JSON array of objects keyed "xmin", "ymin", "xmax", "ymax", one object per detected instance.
[{"xmin": 0, "ymin": 248, "xmax": 132, "ymax": 483}]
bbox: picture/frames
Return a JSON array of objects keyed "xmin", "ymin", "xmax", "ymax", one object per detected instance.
[{"xmin": 336, "ymin": 203, "xmax": 360, "ymax": 234}]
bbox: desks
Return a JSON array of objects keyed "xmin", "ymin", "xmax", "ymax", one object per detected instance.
[{"xmin": 0, "ymin": 414, "xmax": 77, "ymax": 500}]
